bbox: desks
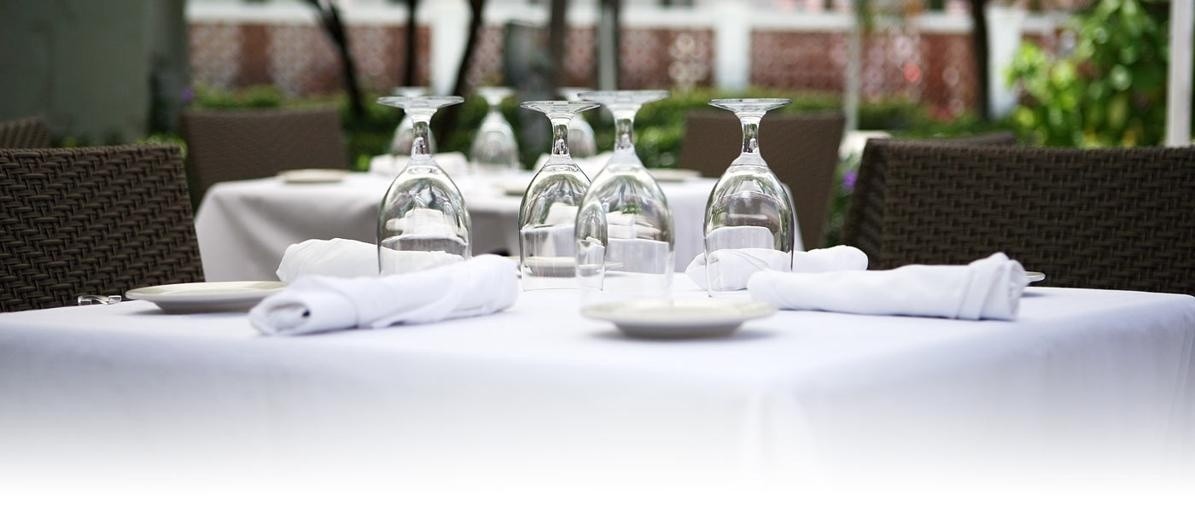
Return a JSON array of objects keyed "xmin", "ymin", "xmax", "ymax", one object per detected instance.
[{"xmin": 191, "ymin": 154, "xmax": 804, "ymax": 284}]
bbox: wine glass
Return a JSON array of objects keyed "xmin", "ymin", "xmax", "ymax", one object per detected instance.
[
  {"xmin": 384, "ymin": 80, "xmax": 440, "ymax": 175},
  {"xmin": 515, "ymin": 99, "xmax": 606, "ymax": 283},
  {"xmin": 554, "ymin": 84, "xmax": 599, "ymax": 178},
  {"xmin": 469, "ymin": 85, "xmax": 521, "ymax": 196},
  {"xmin": 368, "ymin": 92, "xmax": 476, "ymax": 282},
  {"xmin": 572, "ymin": 91, "xmax": 679, "ymax": 284},
  {"xmin": 703, "ymin": 94, "xmax": 800, "ymax": 284}
]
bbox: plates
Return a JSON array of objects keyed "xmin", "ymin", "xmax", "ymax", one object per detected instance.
[
  {"xmin": 582, "ymin": 293, "xmax": 767, "ymax": 345},
  {"xmin": 279, "ymin": 172, "xmax": 347, "ymax": 184},
  {"xmin": 645, "ymin": 165, "xmax": 700, "ymax": 184},
  {"xmin": 120, "ymin": 276, "xmax": 275, "ymax": 313}
]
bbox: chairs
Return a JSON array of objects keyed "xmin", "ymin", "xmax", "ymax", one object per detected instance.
[
  {"xmin": 686, "ymin": 109, "xmax": 841, "ymax": 252},
  {"xmin": 830, "ymin": 136, "xmax": 1195, "ymax": 294},
  {"xmin": 178, "ymin": 108, "xmax": 354, "ymax": 264},
  {"xmin": 0, "ymin": 147, "xmax": 205, "ymax": 310}
]
